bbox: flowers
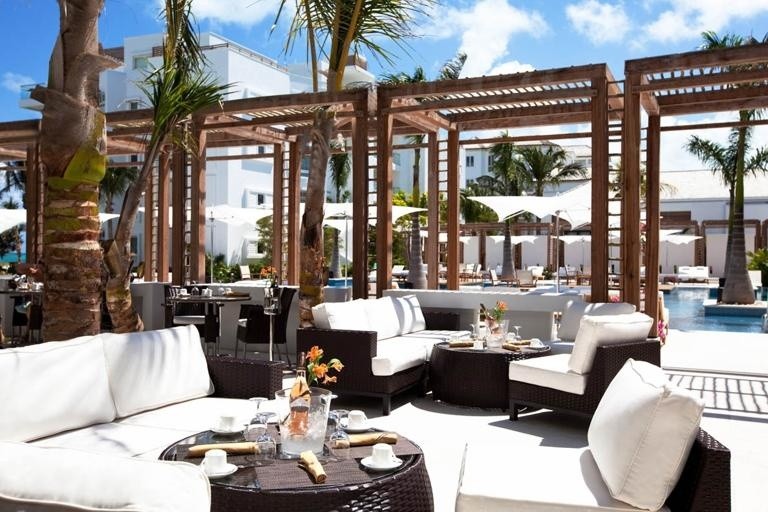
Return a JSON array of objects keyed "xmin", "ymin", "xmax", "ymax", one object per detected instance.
[
  {"xmin": 492, "ymin": 301, "xmax": 508, "ymax": 320},
  {"xmin": 305, "ymin": 345, "xmax": 344, "ymax": 388},
  {"xmin": 261, "ymin": 266, "xmax": 279, "ymax": 283}
]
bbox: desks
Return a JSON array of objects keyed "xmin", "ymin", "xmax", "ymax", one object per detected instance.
[{"xmin": 0, "ymin": 290, "xmax": 42, "ymax": 343}]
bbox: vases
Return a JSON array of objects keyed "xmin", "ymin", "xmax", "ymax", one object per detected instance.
[{"xmin": 484, "ymin": 320, "xmax": 509, "ymax": 347}]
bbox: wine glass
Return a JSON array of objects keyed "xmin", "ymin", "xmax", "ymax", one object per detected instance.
[
  {"xmin": 329, "ymin": 408, "xmax": 350, "ymax": 460},
  {"xmin": 248, "ymin": 397, "xmax": 269, "ymax": 431},
  {"xmin": 255, "ymin": 412, "xmax": 276, "ymax": 465}
]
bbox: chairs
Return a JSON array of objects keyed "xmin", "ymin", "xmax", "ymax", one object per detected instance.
[
  {"xmin": 235, "ymin": 287, "xmax": 297, "ymax": 367},
  {"xmin": 454, "ymin": 358, "xmax": 731, "ymax": 511},
  {"xmin": 508, "ymin": 311, "xmax": 661, "ymax": 422},
  {"xmin": 549, "ymin": 302, "xmax": 638, "ymax": 354},
  {"xmin": 165, "ymin": 284, "xmax": 225, "ymax": 357},
  {"xmin": 393, "ymin": 264, "xmax": 763, "ymax": 299},
  {"xmin": 238, "ymin": 264, "xmax": 263, "ymax": 281}
]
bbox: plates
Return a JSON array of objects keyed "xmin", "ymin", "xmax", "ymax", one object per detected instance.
[
  {"xmin": 344, "ymin": 423, "xmax": 374, "ymax": 431},
  {"xmin": 445, "ymin": 324, "xmax": 545, "ymax": 353},
  {"xmin": 212, "ymin": 426, "xmax": 245, "ymax": 435},
  {"xmin": 200, "ymin": 463, "xmax": 239, "ymax": 479},
  {"xmin": 360, "ymin": 456, "xmax": 404, "ymax": 472}
]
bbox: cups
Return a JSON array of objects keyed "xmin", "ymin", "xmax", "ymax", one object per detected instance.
[
  {"xmin": 179, "ymin": 280, "xmax": 231, "ymax": 299},
  {"xmin": 202, "ymin": 447, "xmax": 227, "ymax": 472},
  {"xmin": 218, "ymin": 413, "xmax": 235, "ymax": 431},
  {"xmin": 347, "ymin": 409, "xmax": 368, "ymax": 427},
  {"xmin": 31, "ymin": 283, "xmax": 40, "ymax": 291},
  {"xmin": 372, "ymin": 444, "xmax": 397, "ymax": 464}
]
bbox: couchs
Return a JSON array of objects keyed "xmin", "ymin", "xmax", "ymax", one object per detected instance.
[
  {"xmin": 0, "ymin": 322, "xmax": 288, "ymax": 512},
  {"xmin": 295, "ymin": 295, "xmax": 473, "ymax": 416}
]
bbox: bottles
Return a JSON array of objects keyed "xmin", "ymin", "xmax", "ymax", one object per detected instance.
[{"xmin": 290, "ymin": 350, "xmax": 310, "ymax": 438}]
